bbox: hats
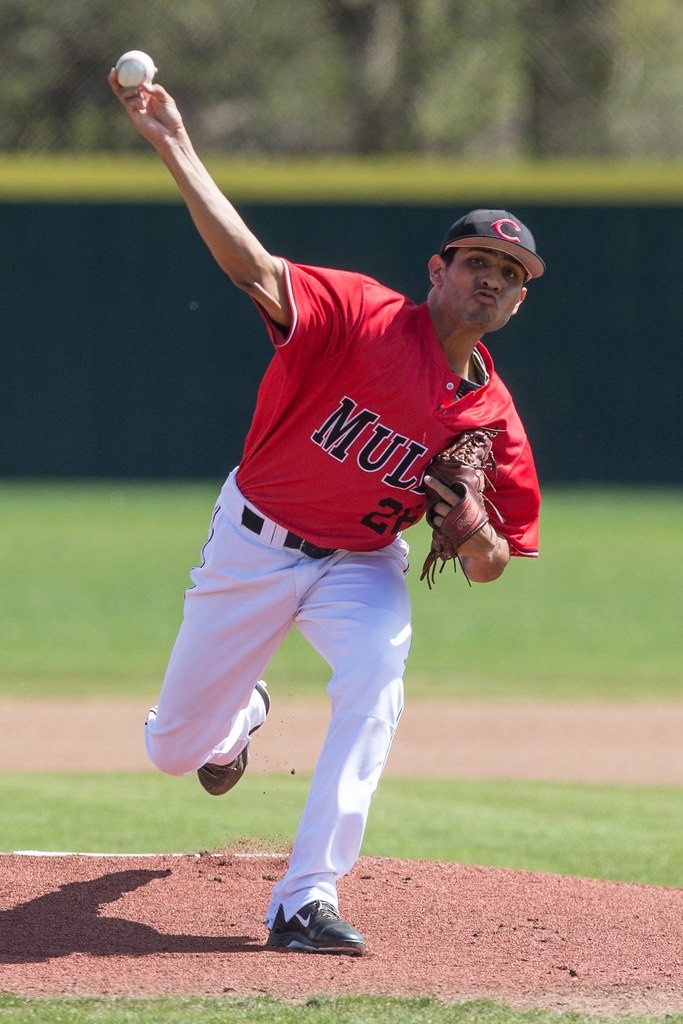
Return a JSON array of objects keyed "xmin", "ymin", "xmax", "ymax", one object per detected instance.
[{"xmin": 441, "ymin": 209, "xmax": 546, "ymax": 285}]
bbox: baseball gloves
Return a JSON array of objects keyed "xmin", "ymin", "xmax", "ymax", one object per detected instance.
[{"xmin": 424, "ymin": 427, "xmax": 491, "ymax": 560}]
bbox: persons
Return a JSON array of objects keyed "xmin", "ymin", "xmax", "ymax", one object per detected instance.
[{"xmin": 108, "ymin": 67, "xmax": 545, "ymax": 956}]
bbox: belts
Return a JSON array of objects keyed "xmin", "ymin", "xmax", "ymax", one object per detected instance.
[{"xmin": 242, "ymin": 507, "xmax": 338, "ymax": 559}]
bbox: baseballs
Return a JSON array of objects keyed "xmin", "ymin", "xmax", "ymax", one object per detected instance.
[{"xmin": 115, "ymin": 50, "xmax": 156, "ymax": 88}]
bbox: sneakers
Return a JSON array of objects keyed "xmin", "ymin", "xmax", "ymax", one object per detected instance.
[
  {"xmin": 267, "ymin": 900, "xmax": 366, "ymax": 956},
  {"xmin": 198, "ymin": 681, "xmax": 271, "ymax": 795}
]
